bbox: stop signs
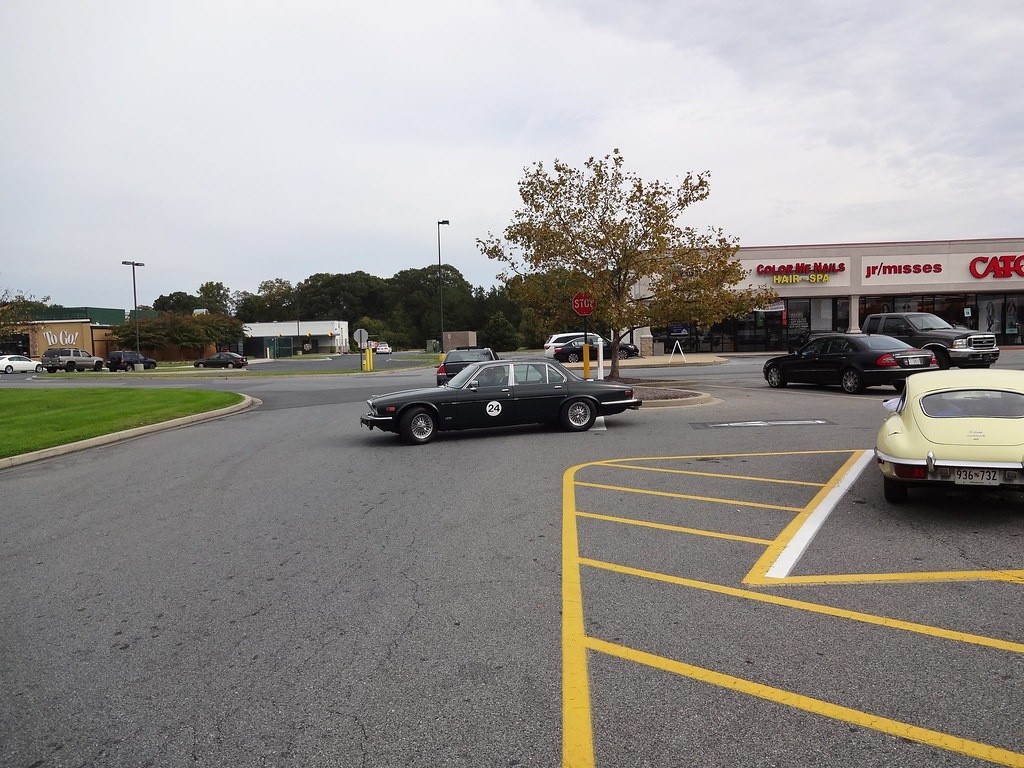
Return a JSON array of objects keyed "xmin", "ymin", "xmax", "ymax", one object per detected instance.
[{"xmin": 572, "ymin": 290, "xmax": 597, "ymax": 316}]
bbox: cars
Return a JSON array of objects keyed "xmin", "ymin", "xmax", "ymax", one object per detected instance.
[
  {"xmin": 0, "ymin": 354, "xmax": 43, "ymax": 374},
  {"xmin": 763, "ymin": 329, "xmax": 940, "ymax": 393},
  {"xmin": 435, "ymin": 346, "xmax": 506, "ymax": 387},
  {"xmin": 376, "ymin": 343, "xmax": 393, "ymax": 354},
  {"xmin": 359, "ymin": 360, "xmax": 643, "ymax": 445},
  {"xmin": 873, "ymin": 368, "xmax": 1024, "ymax": 504},
  {"xmin": 193, "ymin": 352, "xmax": 248, "ymax": 369}
]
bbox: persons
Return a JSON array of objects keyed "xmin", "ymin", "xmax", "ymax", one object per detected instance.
[{"xmin": 493, "ymin": 366, "xmax": 508, "ymax": 385}]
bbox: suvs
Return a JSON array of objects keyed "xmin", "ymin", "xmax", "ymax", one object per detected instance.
[
  {"xmin": 543, "ymin": 332, "xmax": 639, "ymax": 363},
  {"xmin": 41, "ymin": 347, "xmax": 104, "ymax": 373},
  {"xmin": 862, "ymin": 313, "xmax": 999, "ymax": 370},
  {"xmin": 105, "ymin": 350, "xmax": 157, "ymax": 372}
]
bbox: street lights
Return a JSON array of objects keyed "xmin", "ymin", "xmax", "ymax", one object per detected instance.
[
  {"xmin": 122, "ymin": 261, "xmax": 146, "ymax": 364},
  {"xmin": 438, "ymin": 220, "xmax": 449, "ymax": 353}
]
